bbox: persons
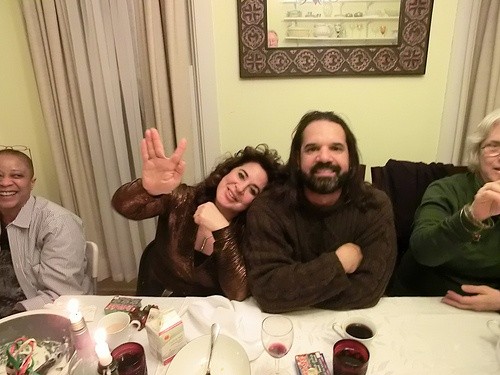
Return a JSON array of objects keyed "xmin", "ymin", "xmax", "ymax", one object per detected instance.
[
  {"xmin": 0, "ymin": 149, "xmax": 94, "ymax": 320},
  {"xmin": 241, "ymin": 110, "xmax": 398, "ymax": 314},
  {"xmin": 388, "ymin": 108, "xmax": 500, "ymax": 313},
  {"xmin": 111, "ymin": 128, "xmax": 286, "ymax": 302}
]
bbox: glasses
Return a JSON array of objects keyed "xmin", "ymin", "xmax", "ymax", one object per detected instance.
[
  {"xmin": 479, "ymin": 144, "xmax": 500, "ymax": 157},
  {"xmin": 0, "ymin": 145, "xmax": 34, "ymax": 176}
]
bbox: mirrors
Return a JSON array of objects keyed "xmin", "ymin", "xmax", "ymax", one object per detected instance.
[{"xmin": 237, "ymin": 0, "xmax": 433, "ymax": 77}]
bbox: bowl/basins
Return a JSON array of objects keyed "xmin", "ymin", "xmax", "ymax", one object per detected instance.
[{"xmin": 1, "ymin": 310, "xmax": 71, "ymax": 375}]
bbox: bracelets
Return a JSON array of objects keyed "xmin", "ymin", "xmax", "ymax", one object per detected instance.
[{"xmin": 460, "ymin": 203, "xmax": 494, "ymax": 242}]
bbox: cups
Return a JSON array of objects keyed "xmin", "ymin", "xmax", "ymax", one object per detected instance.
[
  {"xmin": 109, "ymin": 342, "xmax": 149, "ymax": 375},
  {"xmin": 333, "ymin": 340, "xmax": 370, "ymax": 375},
  {"xmin": 102, "ymin": 312, "xmax": 141, "ymax": 350},
  {"xmin": 331, "ymin": 314, "xmax": 378, "ymax": 346}
]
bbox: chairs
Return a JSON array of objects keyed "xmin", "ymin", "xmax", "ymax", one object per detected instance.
[
  {"xmin": 83, "ymin": 240, "xmax": 98, "ymax": 298},
  {"xmin": 371, "ymin": 159, "xmax": 471, "ymax": 296}
]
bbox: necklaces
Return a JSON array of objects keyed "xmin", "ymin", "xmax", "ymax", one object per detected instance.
[{"xmin": 199, "ymin": 235, "xmax": 213, "ymax": 252}]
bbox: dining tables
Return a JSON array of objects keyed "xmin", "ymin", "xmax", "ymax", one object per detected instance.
[{"xmin": 48, "ymin": 295, "xmax": 500, "ymax": 375}]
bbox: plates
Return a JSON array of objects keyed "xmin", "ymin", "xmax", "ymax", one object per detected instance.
[{"xmin": 163, "ymin": 333, "xmax": 252, "ymax": 375}]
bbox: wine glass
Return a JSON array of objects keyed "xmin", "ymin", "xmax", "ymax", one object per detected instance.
[{"xmin": 262, "ymin": 315, "xmax": 293, "ymax": 375}]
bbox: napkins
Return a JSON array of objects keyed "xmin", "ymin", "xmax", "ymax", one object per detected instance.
[{"xmin": 179, "ymin": 295, "xmax": 259, "ymax": 345}]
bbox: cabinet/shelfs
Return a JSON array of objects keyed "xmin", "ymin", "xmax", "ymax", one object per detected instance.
[{"xmin": 282, "ymin": 13, "xmax": 399, "ymax": 44}]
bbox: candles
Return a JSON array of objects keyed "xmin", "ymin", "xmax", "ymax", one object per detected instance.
[{"xmin": 93, "ymin": 329, "xmax": 113, "ymax": 366}]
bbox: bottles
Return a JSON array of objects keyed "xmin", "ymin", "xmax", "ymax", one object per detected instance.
[{"xmin": 69, "ymin": 314, "xmax": 99, "ymax": 374}]
